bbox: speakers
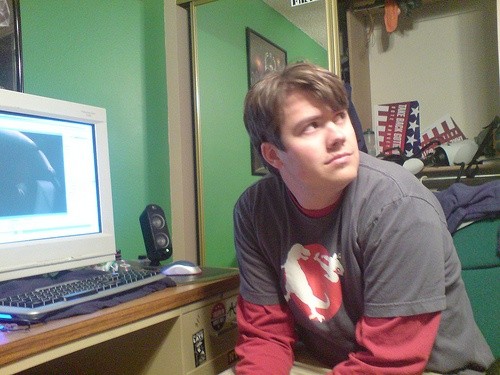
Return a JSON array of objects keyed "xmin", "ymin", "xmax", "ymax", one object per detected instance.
[{"xmin": 139, "ymin": 204, "xmax": 172, "ymax": 269}]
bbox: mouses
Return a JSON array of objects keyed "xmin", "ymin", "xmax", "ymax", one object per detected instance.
[{"xmin": 159, "ymin": 260, "xmax": 202, "ymax": 275}]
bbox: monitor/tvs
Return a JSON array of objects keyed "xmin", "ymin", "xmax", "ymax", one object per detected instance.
[{"xmin": 0, "ymin": 88, "xmax": 117, "ymax": 281}]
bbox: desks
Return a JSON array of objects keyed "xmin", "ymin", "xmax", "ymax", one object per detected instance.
[{"xmin": 0, "ymin": 260, "xmax": 240, "ymax": 375}]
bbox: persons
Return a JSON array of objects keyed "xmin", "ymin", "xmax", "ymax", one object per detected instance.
[{"xmin": 233, "ymin": 63, "xmax": 496, "ymax": 375}]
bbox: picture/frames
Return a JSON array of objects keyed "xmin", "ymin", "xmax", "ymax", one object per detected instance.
[
  {"xmin": 0, "ymin": 0, "xmax": 25, "ymax": 93},
  {"xmin": 245, "ymin": 26, "xmax": 288, "ymax": 176}
]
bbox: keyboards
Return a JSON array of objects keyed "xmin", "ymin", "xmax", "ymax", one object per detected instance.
[{"xmin": 0, "ymin": 270, "xmax": 167, "ymax": 322}]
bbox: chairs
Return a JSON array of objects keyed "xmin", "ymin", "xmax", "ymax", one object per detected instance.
[{"xmin": 432, "ymin": 180, "xmax": 500, "ymax": 360}]
